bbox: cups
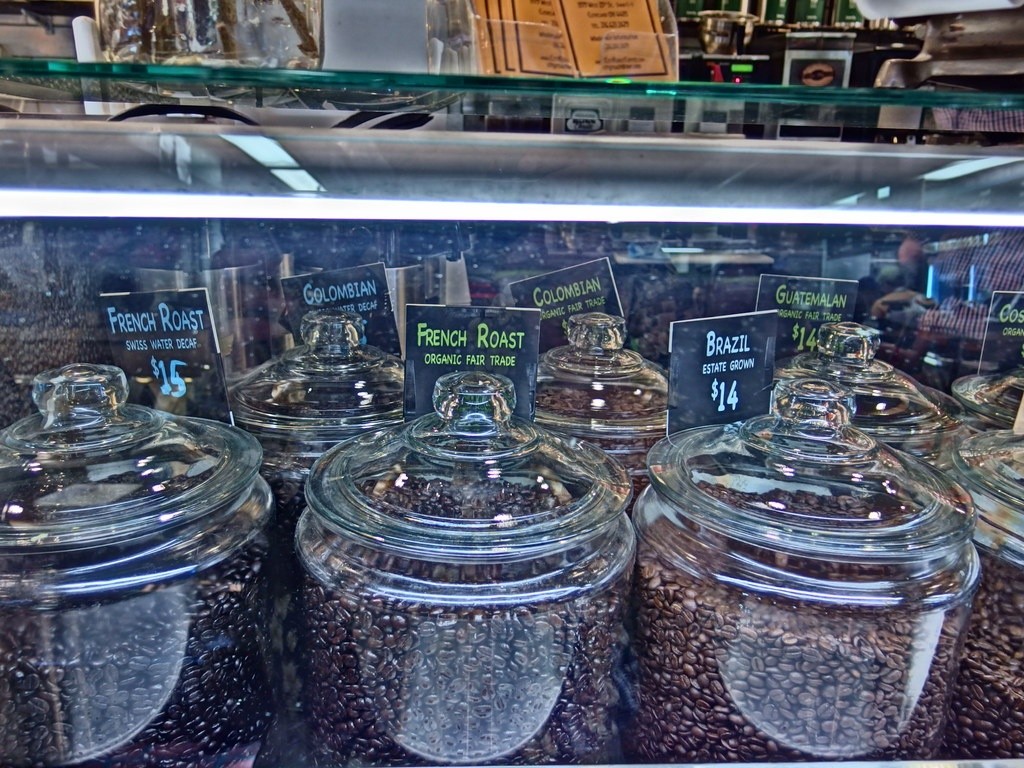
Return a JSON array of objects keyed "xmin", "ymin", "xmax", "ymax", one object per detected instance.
[
  {"xmin": 97, "ymin": 0, "xmax": 324, "ymax": 80},
  {"xmin": 426, "ymin": 0, "xmax": 481, "ymax": 76}
]
